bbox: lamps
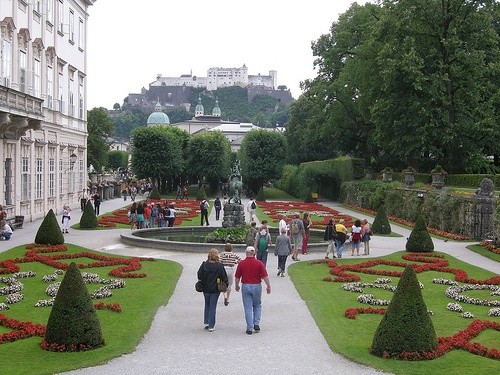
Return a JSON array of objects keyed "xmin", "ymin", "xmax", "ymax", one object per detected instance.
[{"xmin": 66, "ymin": 153, "xmax": 77, "ymax": 173}]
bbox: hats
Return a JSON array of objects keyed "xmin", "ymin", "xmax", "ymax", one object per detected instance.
[
  {"xmin": 281, "ymin": 228, "xmax": 286, "ymax": 233},
  {"xmin": 151, "ymin": 203, "xmax": 154, "ymax": 206},
  {"xmin": 246, "ymin": 246, "xmax": 255, "ymax": 253},
  {"xmin": 295, "ymin": 213, "xmax": 299, "ymax": 217},
  {"xmin": 339, "ymin": 219, "xmax": 344, "ymax": 223}
]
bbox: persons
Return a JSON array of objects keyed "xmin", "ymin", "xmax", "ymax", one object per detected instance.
[
  {"xmin": 200, "ymin": 197, "xmax": 210, "ymax": 226},
  {"xmin": 247, "ymin": 198, "xmax": 257, "ymax": 222},
  {"xmin": 274, "ymin": 228, "xmax": 293, "ymax": 277},
  {"xmin": 234, "ymin": 246, "xmax": 271, "ymax": 335},
  {"xmin": 218, "ymin": 243, "xmax": 241, "ymax": 306},
  {"xmin": 61, "ymin": 204, "xmax": 72, "ymax": 234},
  {"xmin": 325, "ymin": 218, "xmax": 370, "ymax": 259},
  {"xmin": 0, "ymin": 205, "xmax": 13, "ymax": 240},
  {"xmin": 78, "ymin": 189, "xmax": 101, "ymax": 215},
  {"xmin": 197, "ymin": 248, "xmax": 229, "ymax": 331},
  {"xmin": 246, "ymin": 220, "xmax": 272, "ymax": 268},
  {"xmin": 122, "ymin": 181, "xmax": 189, "ymax": 230},
  {"xmin": 279, "ymin": 212, "xmax": 312, "ymax": 261},
  {"xmin": 214, "ymin": 183, "xmax": 228, "ymax": 220}
]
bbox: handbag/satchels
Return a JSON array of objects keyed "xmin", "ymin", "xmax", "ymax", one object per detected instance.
[
  {"xmin": 349, "ymin": 236, "xmax": 353, "ymax": 242},
  {"xmin": 215, "ymin": 206, "xmax": 220, "ymax": 210},
  {"xmin": 196, "ymin": 281, "xmax": 204, "ymax": 292},
  {"xmin": 369, "ymin": 231, "xmax": 373, "ymax": 236}
]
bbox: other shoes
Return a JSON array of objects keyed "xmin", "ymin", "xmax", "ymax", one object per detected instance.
[
  {"xmin": 333, "ymin": 256, "xmax": 337, "ymax": 258},
  {"xmin": 206, "ymin": 324, "xmax": 209, "ymax": 327},
  {"xmin": 292, "ymin": 256, "xmax": 300, "ymax": 261},
  {"xmin": 254, "ymin": 325, "xmax": 260, "ymax": 331},
  {"xmin": 225, "ymin": 302, "xmax": 229, "ymax": 306},
  {"xmin": 281, "ymin": 274, "xmax": 285, "ymax": 276},
  {"xmin": 325, "ymin": 257, "xmax": 330, "ymax": 259},
  {"xmin": 209, "ymin": 327, "xmax": 215, "ymax": 331},
  {"xmin": 277, "ymin": 270, "xmax": 281, "ymax": 275},
  {"xmin": 246, "ymin": 330, "xmax": 252, "ymax": 334}
]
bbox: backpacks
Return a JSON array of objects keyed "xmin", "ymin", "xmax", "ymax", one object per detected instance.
[
  {"xmin": 246, "ymin": 228, "xmax": 256, "ymax": 246},
  {"xmin": 164, "ymin": 209, "xmax": 169, "ymax": 220},
  {"xmin": 291, "ymin": 219, "xmax": 299, "ymax": 233},
  {"xmin": 151, "ymin": 208, "xmax": 157, "ymax": 217},
  {"xmin": 200, "ymin": 200, "xmax": 206, "ymax": 210},
  {"xmin": 251, "ymin": 201, "xmax": 256, "ymax": 209},
  {"xmin": 257, "ymin": 232, "xmax": 268, "ymax": 252}
]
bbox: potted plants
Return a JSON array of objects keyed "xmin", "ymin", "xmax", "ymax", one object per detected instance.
[
  {"xmin": 365, "ymin": 167, "xmax": 373, "ymax": 178},
  {"xmin": 381, "ymin": 167, "xmax": 393, "ymax": 180},
  {"xmin": 431, "ymin": 165, "xmax": 447, "ymax": 187},
  {"xmin": 401, "ymin": 167, "xmax": 416, "ymax": 182}
]
